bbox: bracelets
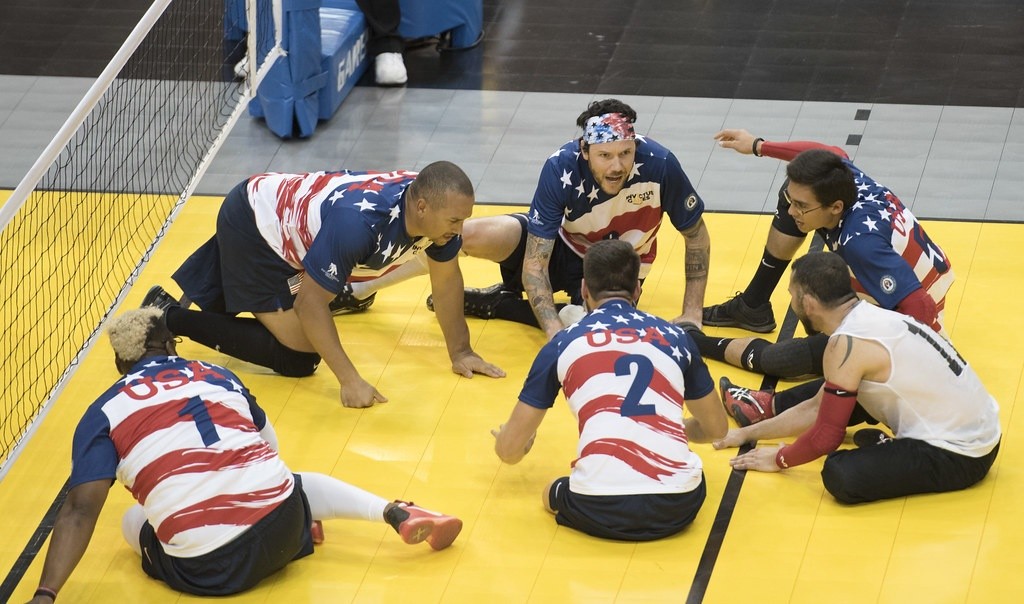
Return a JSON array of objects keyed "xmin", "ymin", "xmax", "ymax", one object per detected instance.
[
  {"xmin": 32, "ymin": 587, "xmax": 60, "ymax": 602},
  {"xmin": 752, "ymin": 136, "xmax": 764, "ymax": 157}
]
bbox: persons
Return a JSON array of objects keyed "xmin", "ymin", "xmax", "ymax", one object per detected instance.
[
  {"xmin": 233, "ymin": 1, "xmax": 411, "ymax": 86},
  {"xmin": 711, "ymin": 251, "xmax": 1002, "ymax": 507},
  {"xmin": 141, "ymin": 159, "xmax": 508, "ymax": 410},
  {"xmin": 330, "ymin": 98, "xmax": 711, "ymax": 339},
  {"xmin": 491, "ymin": 238, "xmax": 729, "ymax": 543},
  {"xmin": 26, "ymin": 305, "xmax": 463, "ymax": 603},
  {"xmin": 672, "ymin": 124, "xmax": 956, "ymax": 378}
]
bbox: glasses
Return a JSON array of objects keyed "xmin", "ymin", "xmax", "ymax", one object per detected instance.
[{"xmin": 783, "ymin": 188, "xmax": 824, "ymax": 216}]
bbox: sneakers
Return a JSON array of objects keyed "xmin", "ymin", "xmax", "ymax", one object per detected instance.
[
  {"xmin": 702, "ymin": 291, "xmax": 777, "ymax": 333},
  {"xmin": 139, "ymin": 284, "xmax": 182, "ymax": 325},
  {"xmin": 674, "ymin": 319, "xmax": 706, "ymax": 336},
  {"xmin": 329, "ymin": 280, "xmax": 377, "ymax": 314},
  {"xmin": 235, "ymin": 58, "xmax": 250, "ymax": 76},
  {"xmin": 428, "ymin": 283, "xmax": 523, "ymax": 320},
  {"xmin": 854, "ymin": 429, "xmax": 893, "ymax": 448},
  {"xmin": 387, "ymin": 500, "xmax": 462, "ymax": 551},
  {"xmin": 376, "ymin": 53, "xmax": 408, "ymax": 84},
  {"xmin": 719, "ymin": 376, "xmax": 774, "ymax": 427},
  {"xmin": 312, "ymin": 521, "xmax": 324, "ymax": 544}
]
